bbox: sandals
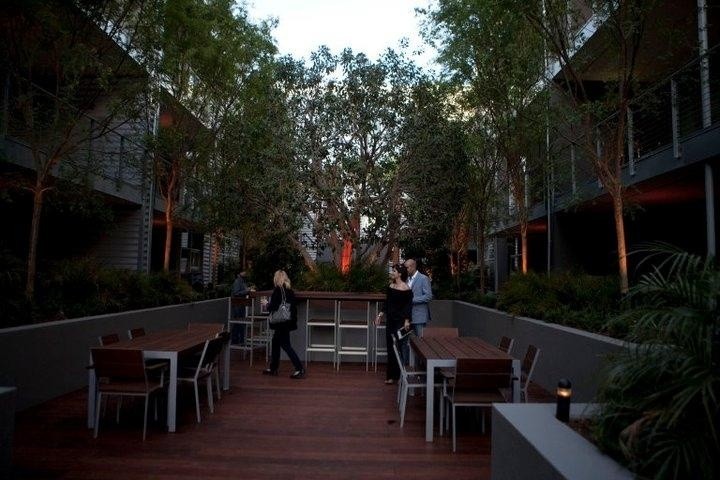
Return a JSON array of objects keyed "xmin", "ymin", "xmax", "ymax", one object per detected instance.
[{"xmin": 385, "ymin": 379, "xmax": 394, "ymax": 385}]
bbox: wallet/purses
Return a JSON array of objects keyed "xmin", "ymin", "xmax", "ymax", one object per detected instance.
[{"xmin": 396, "ymin": 323, "xmax": 414, "ymax": 340}]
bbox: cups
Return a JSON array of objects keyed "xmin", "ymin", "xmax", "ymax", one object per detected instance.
[{"xmin": 260, "ymin": 296, "xmax": 268, "ymax": 305}]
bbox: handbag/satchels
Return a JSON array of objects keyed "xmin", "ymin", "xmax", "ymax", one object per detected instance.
[{"xmin": 268, "ymin": 283, "xmax": 292, "ymax": 325}]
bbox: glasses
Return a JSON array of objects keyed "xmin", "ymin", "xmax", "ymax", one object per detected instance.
[{"xmin": 397, "ymin": 263, "xmax": 403, "ymax": 271}]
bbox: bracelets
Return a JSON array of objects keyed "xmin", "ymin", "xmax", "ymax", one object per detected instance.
[{"xmin": 377, "ymin": 315, "xmax": 382, "ymax": 319}]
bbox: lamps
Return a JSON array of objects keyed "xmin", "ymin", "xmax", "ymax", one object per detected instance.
[{"xmin": 556, "ymin": 377, "xmax": 572, "ymax": 424}]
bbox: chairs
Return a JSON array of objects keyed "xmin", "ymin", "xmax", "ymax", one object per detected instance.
[
  {"xmin": 305, "ymin": 298, "xmax": 337, "ymax": 369},
  {"xmin": 226, "ymin": 293, "xmax": 274, "ymax": 365},
  {"xmin": 420, "ymin": 325, "xmax": 460, "ymax": 337},
  {"xmin": 333, "ymin": 300, "xmax": 371, "ymax": 372},
  {"xmin": 439, "ymin": 357, "xmax": 520, "ymax": 453},
  {"xmin": 389, "ymin": 343, "xmax": 442, "ymax": 428},
  {"xmin": 482, "ymin": 343, "xmax": 540, "ymax": 432},
  {"xmin": 372, "ymin": 301, "xmax": 388, "ymax": 372},
  {"xmin": 85, "ymin": 320, "xmax": 231, "ymax": 439},
  {"xmin": 499, "ymin": 335, "xmax": 515, "ymax": 355},
  {"xmin": 389, "ymin": 332, "xmax": 439, "ymax": 405}
]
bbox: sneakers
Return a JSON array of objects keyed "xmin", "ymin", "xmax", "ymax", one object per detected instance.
[
  {"xmin": 261, "ymin": 368, "xmax": 279, "ymax": 376},
  {"xmin": 289, "ymin": 368, "xmax": 306, "ymax": 379}
]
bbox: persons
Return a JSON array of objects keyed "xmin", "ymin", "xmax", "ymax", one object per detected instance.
[
  {"xmin": 260, "ymin": 268, "xmax": 304, "ymax": 379},
  {"xmin": 374, "ymin": 264, "xmax": 414, "ymax": 384},
  {"xmin": 403, "ymin": 258, "xmax": 433, "ymax": 365},
  {"xmin": 231, "ymin": 270, "xmax": 256, "ymax": 345}
]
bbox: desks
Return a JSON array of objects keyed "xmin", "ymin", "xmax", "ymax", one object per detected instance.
[{"xmin": 407, "ymin": 334, "xmax": 522, "ymax": 443}]
